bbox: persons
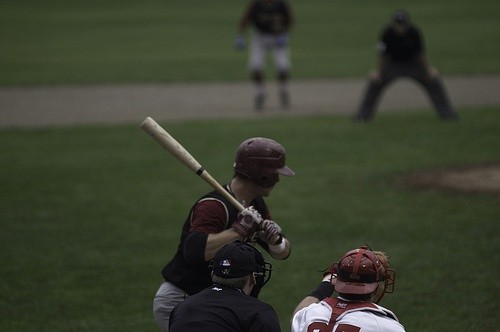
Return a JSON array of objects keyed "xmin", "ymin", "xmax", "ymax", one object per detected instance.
[
  {"xmin": 168, "ymin": 240, "xmax": 281, "ymax": 332},
  {"xmin": 240, "ymin": 0, "xmax": 294, "ymax": 109},
  {"xmin": 351, "ymin": 11, "xmax": 458, "ymax": 121},
  {"xmin": 289, "ymin": 245, "xmax": 406, "ymax": 332},
  {"xmin": 153, "ymin": 137, "xmax": 292, "ymax": 332}
]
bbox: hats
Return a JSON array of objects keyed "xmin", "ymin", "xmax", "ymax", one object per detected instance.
[{"xmin": 212, "ymin": 241, "xmax": 272, "ymax": 289}]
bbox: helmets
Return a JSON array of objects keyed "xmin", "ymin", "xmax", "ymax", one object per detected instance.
[
  {"xmin": 333, "ymin": 245, "xmax": 396, "ymax": 294},
  {"xmin": 232, "ymin": 136, "xmax": 295, "ymax": 176}
]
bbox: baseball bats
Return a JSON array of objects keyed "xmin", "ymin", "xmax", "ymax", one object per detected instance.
[{"xmin": 140, "ymin": 117, "xmax": 282, "ymax": 244}]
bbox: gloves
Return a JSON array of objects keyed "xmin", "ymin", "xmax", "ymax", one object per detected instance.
[
  {"xmin": 261, "ymin": 219, "xmax": 283, "ymax": 247},
  {"xmin": 233, "ymin": 201, "xmax": 262, "ymax": 238}
]
